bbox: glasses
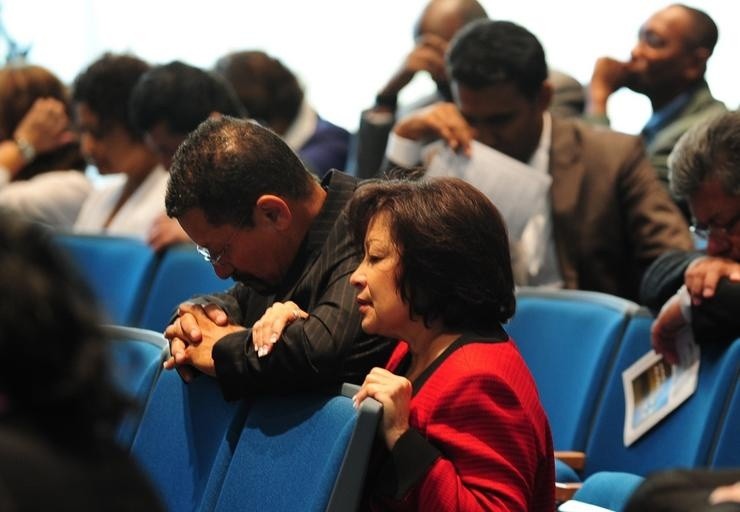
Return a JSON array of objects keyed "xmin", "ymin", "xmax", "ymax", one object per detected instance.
[
  {"xmin": 197, "ymin": 220, "xmax": 242, "ymax": 264},
  {"xmin": 689, "ymin": 212, "xmax": 740, "ymax": 240}
]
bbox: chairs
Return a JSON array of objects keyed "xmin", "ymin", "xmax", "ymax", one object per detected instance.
[
  {"xmin": 206, "ymin": 374, "xmax": 386, "ymax": 511},
  {"xmin": 132, "ymin": 239, "xmax": 243, "ymax": 337},
  {"xmin": 127, "ymin": 343, "xmax": 253, "ymax": 512},
  {"xmin": 555, "ymin": 315, "xmax": 739, "ymax": 512},
  {"xmin": 485, "ymin": 283, "xmax": 658, "ymax": 486},
  {"xmin": 94, "ymin": 321, "xmax": 171, "ymax": 463},
  {"xmin": 50, "ymin": 230, "xmax": 158, "ymax": 335},
  {"xmin": 708, "ymin": 379, "xmax": 739, "ymax": 474}
]
